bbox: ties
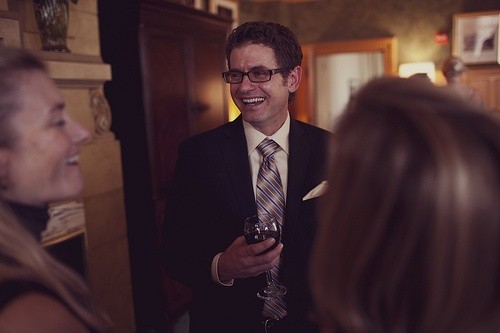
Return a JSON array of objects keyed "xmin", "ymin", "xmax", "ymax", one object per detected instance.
[{"xmin": 255, "ymin": 139, "xmax": 290, "ymax": 322}]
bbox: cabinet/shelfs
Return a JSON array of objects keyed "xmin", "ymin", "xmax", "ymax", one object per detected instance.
[{"xmin": 97, "ymin": 0, "xmax": 235, "ymax": 333}]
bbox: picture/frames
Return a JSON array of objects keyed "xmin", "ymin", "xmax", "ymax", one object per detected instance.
[{"xmin": 451, "ymin": 10, "xmax": 500, "ymax": 66}]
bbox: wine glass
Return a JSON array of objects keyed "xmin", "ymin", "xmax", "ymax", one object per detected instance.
[{"xmin": 242, "ymin": 213, "xmax": 287, "ymax": 299}]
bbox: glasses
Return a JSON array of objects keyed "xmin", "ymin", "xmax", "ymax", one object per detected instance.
[{"xmin": 222, "ymin": 66, "xmax": 284, "ymax": 85}]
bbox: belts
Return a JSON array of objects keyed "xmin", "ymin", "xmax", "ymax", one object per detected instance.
[{"xmin": 259, "ymin": 319, "xmax": 290, "ymax": 333}]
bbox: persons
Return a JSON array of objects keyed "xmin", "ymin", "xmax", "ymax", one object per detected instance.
[
  {"xmin": 0, "ymin": 46, "xmax": 120, "ymax": 333},
  {"xmin": 163, "ymin": 22, "xmax": 335, "ymax": 333},
  {"xmin": 305, "ymin": 57, "xmax": 500, "ymax": 333}
]
